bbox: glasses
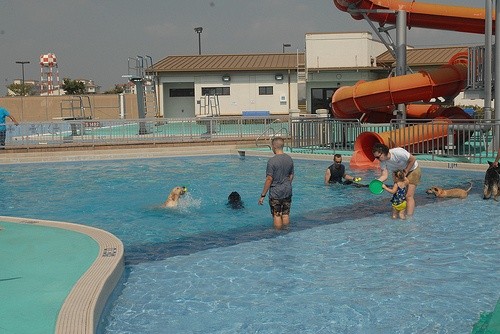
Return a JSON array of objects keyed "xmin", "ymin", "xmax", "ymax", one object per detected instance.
[
  {"xmin": 376, "ymin": 152, "xmax": 383, "ymax": 159},
  {"xmin": 334, "ymin": 160, "xmax": 341, "ymax": 163}
]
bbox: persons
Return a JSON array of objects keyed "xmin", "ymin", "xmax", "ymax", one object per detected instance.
[
  {"xmin": 325, "ymin": 154, "xmax": 369, "ymax": 188},
  {"xmin": 0, "ymin": 106, "xmax": 20, "ymax": 150},
  {"xmin": 258, "ymin": 137, "xmax": 295, "ymax": 232},
  {"xmin": 493, "ymin": 147, "xmax": 500, "ymax": 168},
  {"xmin": 372, "ymin": 143, "xmax": 421, "ymax": 216},
  {"xmin": 382, "ymin": 169, "xmax": 409, "ymax": 220}
]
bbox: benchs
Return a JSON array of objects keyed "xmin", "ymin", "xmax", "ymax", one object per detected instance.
[{"xmin": 242, "ymin": 111, "xmax": 270, "ymax": 125}]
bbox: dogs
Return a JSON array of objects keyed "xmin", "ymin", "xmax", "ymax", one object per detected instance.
[
  {"xmin": 225, "ymin": 191, "xmax": 245, "ymax": 209},
  {"xmin": 145, "ymin": 185, "xmax": 186, "ymax": 210},
  {"xmin": 425, "ymin": 181, "xmax": 473, "ymax": 202},
  {"xmin": 482, "ymin": 160, "xmax": 500, "ymax": 202}
]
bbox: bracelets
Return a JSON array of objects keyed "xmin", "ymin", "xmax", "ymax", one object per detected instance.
[
  {"xmin": 12, "ymin": 119, "xmax": 16, "ymax": 122},
  {"xmin": 261, "ymin": 194, "xmax": 265, "ymax": 197}
]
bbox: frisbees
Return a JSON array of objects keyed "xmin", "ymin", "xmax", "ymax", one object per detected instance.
[{"xmin": 370, "ymin": 179, "xmax": 384, "ymax": 194}]
